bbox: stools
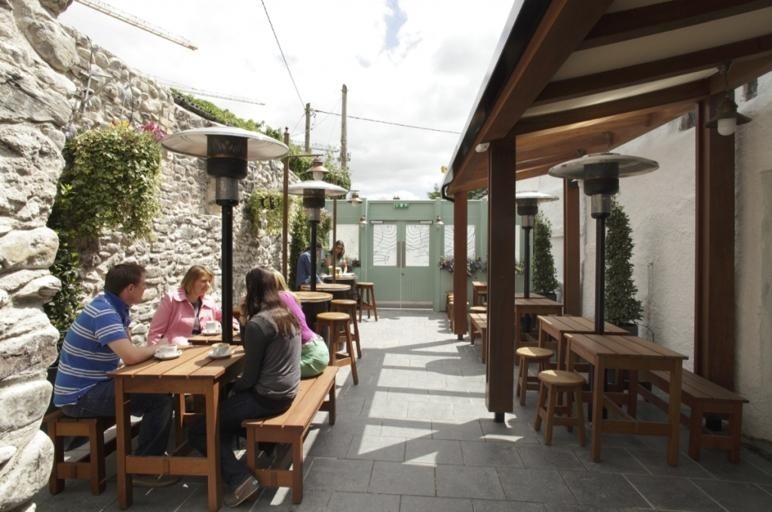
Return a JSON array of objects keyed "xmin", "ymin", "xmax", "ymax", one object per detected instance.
[
  {"xmin": 313, "ymin": 311, "xmax": 359, "ymax": 387},
  {"xmin": 356, "ymin": 281, "xmax": 378, "ymax": 323},
  {"xmin": 514, "ymin": 344, "xmax": 555, "ymax": 407},
  {"xmin": 331, "ymin": 298, "xmax": 362, "ymax": 358},
  {"xmin": 533, "ymin": 369, "xmax": 589, "ymax": 449}
]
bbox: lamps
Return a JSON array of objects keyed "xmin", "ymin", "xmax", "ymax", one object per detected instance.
[{"xmin": 701, "ymin": 55, "xmax": 754, "ymax": 140}]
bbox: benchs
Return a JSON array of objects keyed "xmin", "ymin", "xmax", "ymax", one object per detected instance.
[
  {"xmin": 240, "ymin": 365, "xmax": 338, "ymax": 505},
  {"xmin": 42, "ymin": 387, "xmax": 174, "ymax": 497},
  {"xmin": 613, "ymin": 366, "xmax": 749, "ymax": 470}
]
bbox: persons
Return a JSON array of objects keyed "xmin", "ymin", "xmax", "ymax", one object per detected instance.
[
  {"xmin": 53, "ymin": 264, "xmax": 180, "ymax": 486},
  {"xmin": 233, "ymin": 265, "xmax": 330, "ymax": 378},
  {"xmin": 324, "ymin": 241, "xmax": 347, "ymax": 275},
  {"xmin": 148, "ymin": 265, "xmax": 239, "ymax": 454},
  {"xmin": 188, "ymin": 269, "xmax": 301, "ymax": 509},
  {"xmin": 295, "ymin": 243, "xmax": 323, "ymax": 291}
]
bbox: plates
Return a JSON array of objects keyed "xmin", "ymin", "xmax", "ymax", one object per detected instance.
[
  {"xmin": 201, "ymin": 329, "xmax": 220, "ymax": 336},
  {"xmin": 205, "ymin": 350, "xmax": 235, "ymax": 359},
  {"xmin": 153, "ymin": 350, "xmax": 182, "ymax": 360}
]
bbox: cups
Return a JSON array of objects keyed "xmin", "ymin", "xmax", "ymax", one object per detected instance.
[
  {"xmin": 211, "ymin": 342, "xmax": 230, "ymax": 355},
  {"xmin": 335, "ymin": 267, "xmax": 341, "ymax": 276},
  {"xmin": 205, "ymin": 321, "xmax": 221, "ymax": 332},
  {"xmin": 328, "ymin": 265, "xmax": 333, "ymax": 275},
  {"xmin": 154, "ymin": 344, "xmax": 179, "ymax": 357}
]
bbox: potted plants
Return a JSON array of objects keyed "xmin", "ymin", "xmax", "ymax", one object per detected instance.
[
  {"xmin": 239, "ymin": 186, "xmax": 295, "ymax": 250},
  {"xmin": 530, "ymin": 211, "xmax": 560, "ymax": 339},
  {"xmin": 583, "ymin": 189, "xmax": 645, "ymax": 388}
]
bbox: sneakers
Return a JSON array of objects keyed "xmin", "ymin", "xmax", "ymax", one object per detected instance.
[
  {"xmin": 132, "ymin": 475, "xmax": 175, "ymax": 487},
  {"xmin": 225, "ymin": 476, "xmax": 259, "ymax": 508}
]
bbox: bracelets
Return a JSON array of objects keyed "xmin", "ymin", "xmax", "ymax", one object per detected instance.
[{"xmin": 240, "ymin": 314, "xmax": 245, "ymax": 317}]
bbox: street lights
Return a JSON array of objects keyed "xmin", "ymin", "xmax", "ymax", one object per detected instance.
[
  {"xmin": 278, "ymin": 127, "xmax": 331, "ymax": 284},
  {"xmin": 329, "ymin": 171, "xmax": 365, "ymax": 285}
]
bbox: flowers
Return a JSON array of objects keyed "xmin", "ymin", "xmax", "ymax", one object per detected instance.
[{"xmin": 62, "ymin": 110, "xmax": 168, "ymax": 157}]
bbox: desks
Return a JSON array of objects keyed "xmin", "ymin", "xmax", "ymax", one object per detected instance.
[
  {"xmin": 444, "ymin": 275, "xmax": 567, "ymax": 363},
  {"xmin": 104, "ymin": 330, "xmax": 251, "ymax": 509},
  {"xmin": 228, "ymin": 263, "xmax": 356, "ymax": 329},
  {"xmin": 537, "ymin": 313, "xmax": 633, "ymax": 403},
  {"xmin": 563, "ymin": 332, "xmax": 690, "ymax": 466}
]
